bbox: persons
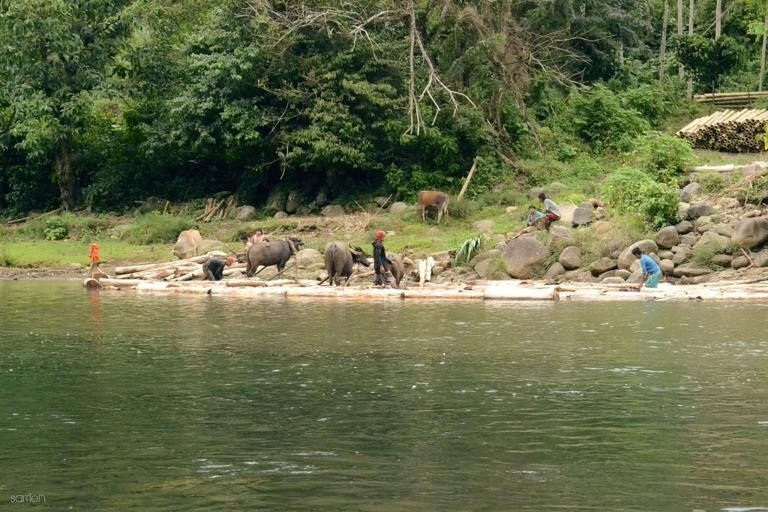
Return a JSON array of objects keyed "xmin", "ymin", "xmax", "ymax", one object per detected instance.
[
  {"xmin": 253, "ymin": 228, "xmax": 270, "ymax": 245},
  {"xmin": 202, "ymin": 256, "xmax": 234, "ymax": 281},
  {"xmin": 240, "ymin": 234, "xmax": 252, "ymax": 253},
  {"xmin": 594, "ymin": 204, "xmax": 605, "ymax": 222},
  {"xmin": 631, "ymin": 247, "xmax": 660, "ymax": 288},
  {"xmin": 512, "ymin": 204, "xmax": 547, "ymax": 239},
  {"xmin": 538, "ymin": 192, "xmax": 562, "ymax": 233},
  {"xmin": 371, "ymin": 231, "xmax": 400, "ymax": 288}
]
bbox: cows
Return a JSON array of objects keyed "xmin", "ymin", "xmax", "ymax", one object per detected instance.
[
  {"xmin": 418, "ymin": 190, "xmax": 449, "ymax": 224},
  {"xmin": 324, "ymin": 241, "xmax": 371, "ymax": 286},
  {"xmin": 173, "ymin": 229, "xmax": 202, "ymax": 260},
  {"xmin": 246, "ymin": 236, "xmax": 304, "ymax": 277},
  {"xmin": 382, "ymin": 251, "xmax": 413, "ymax": 288}
]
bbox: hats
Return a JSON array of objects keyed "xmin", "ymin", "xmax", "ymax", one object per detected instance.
[{"xmin": 376, "ymin": 231, "xmax": 385, "ymax": 238}]
